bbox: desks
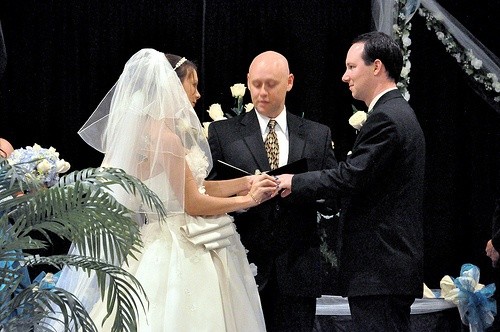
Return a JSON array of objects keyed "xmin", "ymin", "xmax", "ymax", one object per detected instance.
[{"xmin": 314, "ymin": 288, "xmax": 457, "ymax": 332}]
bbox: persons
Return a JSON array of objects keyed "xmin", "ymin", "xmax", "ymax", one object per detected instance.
[
  {"xmin": 118, "ymin": 48, "xmax": 281, "ymax": 332},
  {"xmin": 203, "ymin": 51, "xmax": 332, "ymax": 332},
  {"xmin": 267, "ymin": 31, "xmax": 425, "ymax": 332},
  {"xmin": 0, "ymin": 137, "xmax": 47, "ymax": 332}
]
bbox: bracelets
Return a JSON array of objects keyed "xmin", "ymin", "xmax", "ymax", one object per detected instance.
[{"xmin": 248, "ymin": 192, "xmax": 261, "ymax": 205}]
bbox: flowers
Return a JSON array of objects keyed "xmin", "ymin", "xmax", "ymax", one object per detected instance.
[
  {"xmin": 393, "ymin": 0, "xmax": 500, "ymax": 103},
  {"xmin": 203, "ymin": 83, "xmax": 255, "ymax": 138},
  {"xmin": 349, "ymin": 104, "xmax": 374, "ymax": 131},
  {"xmin": 6, "ymin": 142, "xmax": 70, "ymax": 187}
]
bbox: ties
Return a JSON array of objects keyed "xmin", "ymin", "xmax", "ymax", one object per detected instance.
[{"xmin": 264, "ymin": 119, "xmax": 280, "ymax": 171}]
